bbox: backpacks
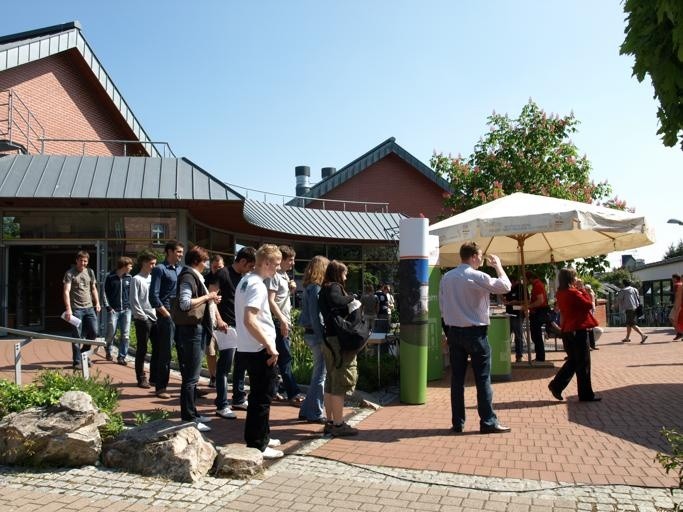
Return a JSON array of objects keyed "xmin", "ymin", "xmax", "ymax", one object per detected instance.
[
  {"xmin": 319, "ymin": 282, "xmax": 372, "ymax": 369},
  {"xmin": 635, "ymin": 304, "xmax": 645, "ymax": 319}
]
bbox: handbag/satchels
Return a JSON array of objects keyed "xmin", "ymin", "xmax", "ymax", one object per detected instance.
[{"xmin": 374, "ymin": 319, "xmax": 390, "ymax": 333}]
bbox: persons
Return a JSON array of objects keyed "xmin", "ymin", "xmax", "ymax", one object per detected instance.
[
  {"xmin": 619, "ymin": 279, "xmax": 648, "ymax": 343},
  {"xmin": 525, "ymin": 271, "xmax": 547, "ymax": 365},
  {"xmin": 439, "ymin": 241, "xmax": 514, "ymax": 433},
  {"xmin": 234, "ymin": 243, "xmax": 284, "ymax": 458},
  {"xmin": 500, "ymin": 274, "xmax": 530, "ymax": 362},
  {"xmin": 148, "ymin": 240, "xmax": 185, "ymax": 398},
  {"xmin": 583, "ymin": 284, "xmax": 603, "ymax": 351},
  {"xmin": 128, "ymin": 250, "xmax": 158, "ymax": 388},
  {"xmin": 673, "ymin": 275, "xmax": 683, "ymax": 341},
  {"xmin": 206, "ymin": 246, "xmax": 257, "ymax": 419},
  {"xmin": 63, "ymin": 251, "xmax": 101, "ymax": 376},
  {"xmin": 669, "ymin": 273, "xmax": 682, "ymax": 331},
  {"xmin": 299, "ymin": 256, "xmax": 331, "ymax": 425},
  {"xmin": 173, "ymin": 245, "xmax": 222, "ymax": 431},
  {"xmin": 318, "ymin": 259, "xmax": 359, "ymax": 437},
  {"xmin": 375, "ymin": 283, "xmax": 394, "ymax": 328},
  {"xmin": 384, "ymin": 285, "xmax": 394, "ymax": 325},
  {"xmin": 548, "ymin": 268, "xmax": 602, "ymax": 401},
  {"xmin": 264, "ymin": 245, "xmax": 307, "ymax": 407},
  {"xmin": 544, "ymin": 301, "xmax": 563, "ymax": 339},
  {"xmin": 102, "ymin": 256, "xmax": 134, "ymax": 365},
  {"xmin": 204, "ymin": 255, "xmax": 225, "ymax": 388},
  {"xmin": 359, "ymin": 283, "xmax": 379, "ymax": 333}
]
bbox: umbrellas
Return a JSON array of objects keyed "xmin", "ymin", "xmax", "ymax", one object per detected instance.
[{"xmin": 427, "ymin": 189, "xmax": 655, "ymax": 367}]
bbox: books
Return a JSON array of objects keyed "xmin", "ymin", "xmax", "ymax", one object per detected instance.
[{"xmin": 61, "ymin": 312, "xmax": 82, "ymax": 328}]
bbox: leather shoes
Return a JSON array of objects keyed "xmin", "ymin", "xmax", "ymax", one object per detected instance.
[
  {"xmin": 452, "ymin": 424, "xmax": 464, "ymax": 433},
  {"xmin": 548, "ymin": 383, "xmax": 564, "ymax": 401},
  {"xmin": 480, "ymin": 423, "xmax": 511, "ymax": 434},
  {"xmin": 580, "ymin": 394, "xmax": 603, "ymax": 402}
]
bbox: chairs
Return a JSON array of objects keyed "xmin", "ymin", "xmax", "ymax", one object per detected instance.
[{"xmin": 544, "ymin": 312, "xmax": 565, "ymax": 351}]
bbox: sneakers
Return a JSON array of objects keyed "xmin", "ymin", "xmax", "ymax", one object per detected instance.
[
  {"xmin": 268, "ymin": 437, "xmax": 281, "ymax": 447},
  {"xmin": 272, "ymin": 392, "xmax": 289, "ymax": 404},
  {"xmin": 262, "ymin": 446, "xmax": 284, "ymax": 458},
  {"xmin": 196, "ymin": 416, "xmax": 211, "ymax": 422},
  {"xmin": 324, "ymin": 421, "xmax": 358, "ymax": 437},
  {"xmin": 673, "ymin": 334, "xmax": 681, "ymax": 340},
  {"xmin": 157, "ymin": 389, "xmax": 170, "ymax": 398},
  {"xmin": 73, "ymin": 363, "xmax": 82, "ymax": 370},
  {"xmin": 215, "ymin": 408, "xmax": 237, "ymax": 420},
  {"xmin": 232, "ymin": 400, "xmax": 248, "ymax": 411},
  {"xmin": 209, "ymin": 377, "xmax": 216, "ymax": 387},
  {"xmin": 640, "ymin": 334, "xmax": 648, "ymax": 344},
  {"xmin": 196, "ymin": 389, "xmax": 208, "ymax": 398},
  {"xmin": 299, "ymin": 411, "xmax": 308, "ymax": 420},
  {"xmin": 622, "ymin": 336, "xmax": 631, "ymax": 342},
  {"xmin": 290, "ymin": 396, "xmax": 304, "ymax": 406},
  {"xmin": 117, "ymin": 357, "xmax": 127, "ymax": 365},
  {"xmin": 182, "ymin": 420, "xmax": 211, "ymax": 432},
  {"xmin": 138, "ymin": 377, "xmax": 151, "ymax": 389},
  {"xmin": 106, "ymin": 353, "xmax": 113, "ymax": 361},
  {"xmin": 307, "ymin": 417, "xmax": 328, "ymax": 425}
]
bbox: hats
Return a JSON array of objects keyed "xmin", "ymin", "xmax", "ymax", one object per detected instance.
[{"xmin": 525, "ymin": 271, "xmax": 532, "ymax": 278}]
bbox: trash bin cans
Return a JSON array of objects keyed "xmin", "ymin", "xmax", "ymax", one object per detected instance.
[{"xmin": 488, "ymin": 313, "xmax": 513, "ymax": 380}]
